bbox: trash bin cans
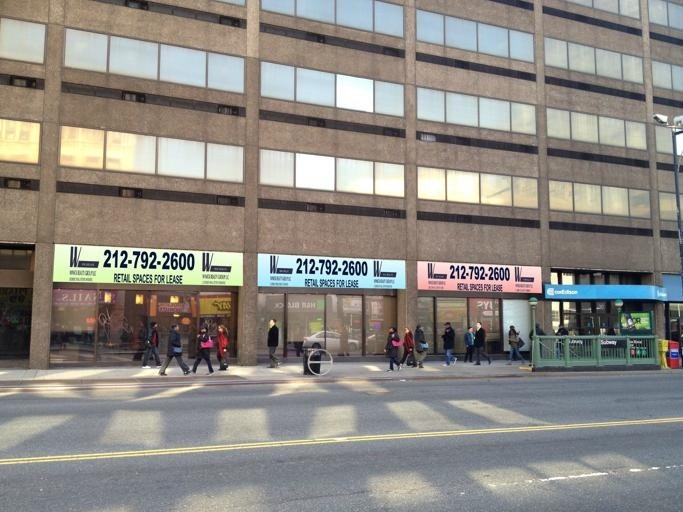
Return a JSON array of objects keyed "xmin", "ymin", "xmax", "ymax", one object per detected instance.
[{"xmin": 304, "ymin": 348, "xmax": 321, "ymax": 376}]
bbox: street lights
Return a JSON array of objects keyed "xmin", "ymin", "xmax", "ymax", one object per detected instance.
[
  {"xmin": 652, "ymin": 111, "xmax": 683, "ymax": 285},
  {"xmin": 614, "ymin": 298, "xmax": 624, "ymax": 336},
  {"xmin": 528, "ymin": 296, "xmax": 537, "ymax": 337}
]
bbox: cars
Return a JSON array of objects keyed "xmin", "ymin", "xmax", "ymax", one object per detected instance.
[
  {"xmin": 302, "ymin": 329, "xmax": 360, "ymax": 356},
  {"xmin": 363, "ymin": 331, "xmax": 387, "ymax": 354}
]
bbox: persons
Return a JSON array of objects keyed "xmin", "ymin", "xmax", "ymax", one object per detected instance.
[
  {"xmin": 190, "ymin": 323, "xmax": 215, "ymax": 376},
  {"xmin": 267, "ymin": 319, "xmax": 282, "ymax": 368},
  {"xmin": 216, "ymin": 324, "xmax": 229, "ymax": 370},
  {"xmin": 384, "ymin": 323, "xmax": 568, "ymax": 373},
  {"xmin": 158, "ymin": 324, "xmax": 191, "ymax": 376},
  {"xmin": 141, "ymin": 321, "xmax": 161, "ymax": 369}
]
